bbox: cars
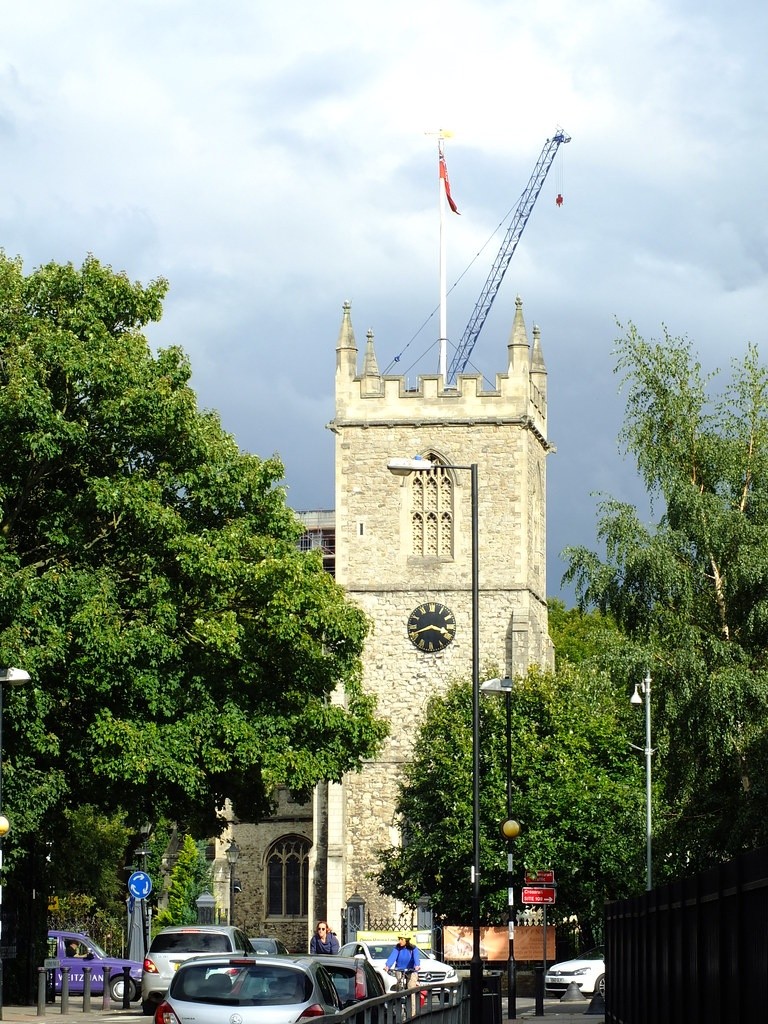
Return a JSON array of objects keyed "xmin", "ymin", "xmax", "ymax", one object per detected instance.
[
  {"xmin": 48, "ymin": 930, "xmax": 143, "ymax": 1003},
  {"xmin": 231, "ymin": 953, "xmax": 396, "ymax": 1024},
  {"xmin": 143, "ymin": 926, "xmax": 257, "ymax": 1014},
  {"xmin": 249, "ymin": 938, "xmax": 289, "ymax": 955},
  {"xmin": 153, "ymin": 955, "xmax": 360, "ymax": 1024},
  {"xmin": 544, "ymin": 945, "xmax": 605, "ymax": 997},
  {"xmin": 338, "ymin": 941, "xmax": 459, "ymax": 1002}
]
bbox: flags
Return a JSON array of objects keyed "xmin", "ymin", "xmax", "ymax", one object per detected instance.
[{"xmin": 437, "ymin": 150, "xmax": 461, "ymax": 217}]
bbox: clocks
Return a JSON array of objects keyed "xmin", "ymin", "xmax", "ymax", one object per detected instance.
[{"xmin": 408, "ymin": 602, "xmax": 458, "ymax": 653}]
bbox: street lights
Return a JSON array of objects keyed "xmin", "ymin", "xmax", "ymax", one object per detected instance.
[
  {"xmin": 477, "ymin": 675, "xmax": 517, "ymax": 1019},
  {"xmin": 388, "ymin": 457, "xmax": 483, "ymax": 1024},
  {"xmin": 631, "ymin": 683, "xmax": 652, "ymax": 892},
  {"xmin": 134, "ymin": 821, "xmax": 152, "ymax": 954},
  {"xmin": 224, "ymin": 837, "xmax": 241, "ymax": 926}
]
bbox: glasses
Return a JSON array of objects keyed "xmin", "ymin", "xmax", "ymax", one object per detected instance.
[{"xmin": 317, "ymin": 927, "xmax": 324, "ymax": 931}]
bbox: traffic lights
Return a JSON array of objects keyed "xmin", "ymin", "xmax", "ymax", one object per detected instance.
[{"xmin": 106, "ymin": 931, "xmax": 112, "ymax": 944}]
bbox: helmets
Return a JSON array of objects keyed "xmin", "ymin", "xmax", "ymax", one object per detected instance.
[{"xmin": 399, "ymin": 933, "xmax": 410, "ymax": 938}]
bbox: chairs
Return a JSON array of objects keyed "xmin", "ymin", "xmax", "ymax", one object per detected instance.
[
  {"xmin": 269, "ymin": 981, "xmax": 296, "ymax": 999},
  {"xmin": 203, "ymin": 974, "xmax": 233, "ymax": 996}
]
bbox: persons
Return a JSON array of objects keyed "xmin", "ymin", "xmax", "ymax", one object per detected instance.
[
  {"xmin": 383, "ymin": 932, "xmax": 420, "ymax": 1022},
  {"xmin": 309, "ymin": 921, "xmax": 339, "ymax": 956},
  {"xmin": 66, "ymin": 941, "xmax": 88, "ymax": 958}
]
